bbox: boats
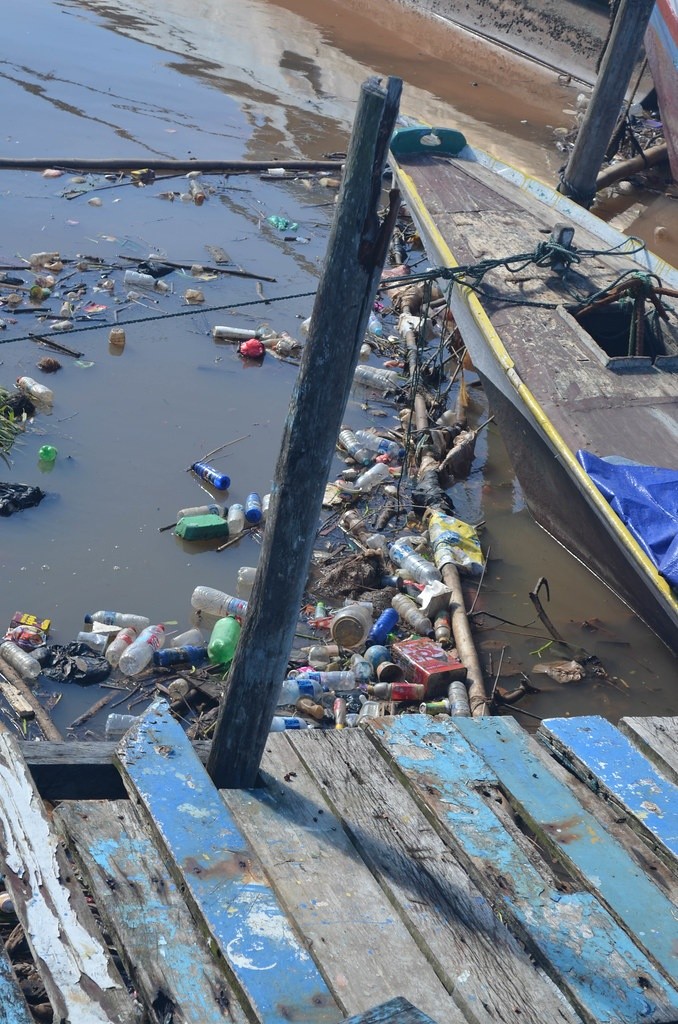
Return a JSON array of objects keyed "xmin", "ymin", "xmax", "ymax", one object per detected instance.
[{"xmin": 382, "ymin": 106, "xmax": 677, "ymax": 627}]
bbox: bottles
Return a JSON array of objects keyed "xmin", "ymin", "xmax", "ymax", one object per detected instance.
[
  {"xmin": 124, "ymin": 270, "xmax": 158, "ymax": 288},
  {"xmin": 360, "ymin": 343, "xmax": 371, "ymax": 356},
  {"xmin": 213, "ymin": 316, "xmax": 311, "ymax": 362},
  {"xmin": 0, "ymin": 427, "xmax": 472, "ymax": 738},
  {"xmin": 189, "ymin": 179, "xmax": 204, "ymax": 201},
  {"xmin": 108, "ymin": 328, "xmax": 125, "ymax": 345},
  {"xmin": 17, "ymin": 376, "xmax": 53, "ymax": 401},
  {"xmin": 357, "ymin": 364, "xmax": 398, "ymax": 383},
  {"xmin": 31, "ymin": 252, "xmax": 59, "ymax": 268},
  {"xmin": 367, "ymin": 311, "xmax": 383, "ymax": 336},
  {"xmin": 352, "ymin": 368, "xmax": 393, "ymax": 392}
]
